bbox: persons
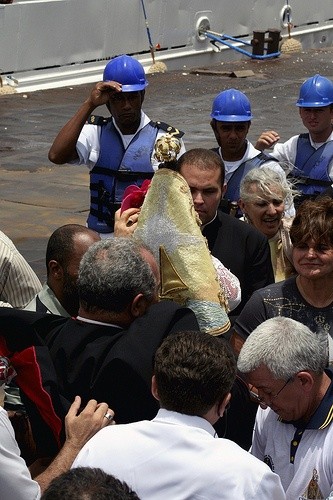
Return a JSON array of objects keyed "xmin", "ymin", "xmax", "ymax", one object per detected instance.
[{"xmin": 0, "ymin": 53, "xmax": 333, "ymax": 500}]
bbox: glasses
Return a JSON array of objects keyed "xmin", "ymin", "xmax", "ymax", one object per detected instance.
[{"xmin": 250, "ymin": 369, "xmax": 309, "ymax": 407}]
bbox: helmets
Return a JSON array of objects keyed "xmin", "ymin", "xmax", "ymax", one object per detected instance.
[
  {"xmin": 296, "ymin": 74, "xmax": 333, "ymax": 107},
  {"xmin": 103, "ymin": 54, "xmax": 149, "ymax": 92},
  {"xmin": 209, "ymin": 88, "xmax": 252, "ymax": 123}
]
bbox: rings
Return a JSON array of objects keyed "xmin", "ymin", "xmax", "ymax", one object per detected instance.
[{"xmin": 104, "ymin": 413, "xmax": 113, "ymax": 421}]
